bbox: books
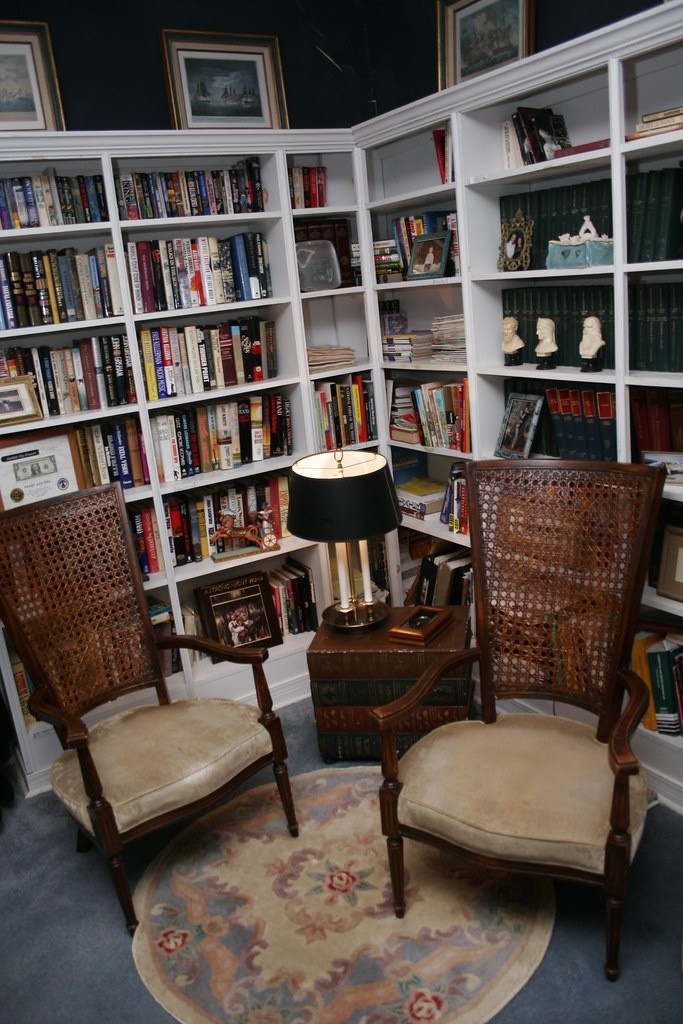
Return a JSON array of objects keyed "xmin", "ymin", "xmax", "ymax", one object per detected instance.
[
  {"xmin": 394, "ymin": 459, "xmax": 470, "ymax": 536},
  {"xmin": 76, "ymin": 392, "xmax": 293, "ymax": 491},
  {"xmin": 488, "ymin": 576, "xmax": 683, "ymax": 739},
  {"xmin": 287, "ymin": 166, "xmax": 327, "ymax": 209},
  {"xmin": 386, "ymin": 378, "xmax": 471, "ymax": 454},
  {"xmin": 10, "ymin": 593, "xmax": 210, "ymax": 727},
  {"xmin": 628, "ymin": 282, "xmax": 683, "ymax": 372},
  {"xmin": 378, "ymin": 298, "xmax": 467, "ymax": 365},
  {"xmin": 307, "ymin": 344, "xmax": 355, "ymax": 374},
  {"xmin": 501, "ymin": 284, "xmax": 615, "ymax": 370},
  {"xmin": 0, "ymin": 157, "xmax": 265, "ymax": 230},
  {"xmin": 544, "ymin": 385, "xmax": 616, "ymax": 462},
  {"xmin": 626, "ymin": 167, "xmax": 683, "ymax": 264},
  {"xmin": 502, "ymin": 106, "xmax": 611, "ymax": 170},
  {"xmin": 0, "ymin": 232, "xmax": 273, "ymax": 334},
  {"xmin": 267, "ymin": 556, "xmax": 319, "ymax": 636},
  {"xmin": 293, "ymin": 209, "xmax": 462, "ymax": 290},
  {"xmin": 0, "ymin": 316, "xmax": 278, "ymax": 414},
  {"xmin": 432, "ymin": 121, "xmax": 455, "ymax": 185},
  {"xmin": 403, "ymin": 548, "xmax": 477, "ymax": 635},
  {"xmin": 499, "ymin": 178, "xmax": 613, "ymax": 271},
  {"xmin": 310, "ymin": 373, "xmax": 378, "ymax": 452},
  {"xmin": 625, "ymin": 105, "xmax": 683, "ymax": 142},
  {"xmin": 124, "ymin": 471, "xmax": 294, "ymax": 575},
  {"xmin": 630, "ymin": 388, "xmax": 683, "ymax": 463}
]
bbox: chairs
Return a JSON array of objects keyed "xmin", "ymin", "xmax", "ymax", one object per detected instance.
[
  {"xmin": 366, "ymin": 461, "xmax": 667, "ymax": 983},
  {"xmin": 0, "ymin": 480, "xmax": 298, "ymax": 933}
]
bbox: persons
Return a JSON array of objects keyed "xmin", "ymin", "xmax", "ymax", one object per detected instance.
[
  {"xmin": 579, "ymin": 316, "xmax": 605, "ymax": 357},
  {"xmin": 501, "ymin": 316, "xmax": 523, "ymax": 352},
  {"xmin": 507, "ymin": 233, "xmax": 516, "ymax": 259},
  {"xmin": 535, "ymin": 318, "xmax": 558, "ymax": 357},
  {"xmin": 513, "ymin": 237, "xmax": 523, "ymax": 259}
]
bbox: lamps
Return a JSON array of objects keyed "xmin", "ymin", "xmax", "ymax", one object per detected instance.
[{"xmin": 284, "ymin": 444, "xmax": 404, "ymax": 636}]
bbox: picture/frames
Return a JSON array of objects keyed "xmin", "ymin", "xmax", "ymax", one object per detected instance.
[
  {"xmin": 0, "ymin": 375, "xmax": 43, "ymax": 427},
  {"xmin": 494, "ymin": 393, "xmax": 545, "ymax": 459},
  {"xmin": 496, "ymin": 207, "xmax": 534, "ymax": 271},
  {"xmin": 437, "ymin": 0, "xmax": 537, "ymax": 92},
  {"xmin": 197, "ymin": 570, "xmax": 284, "ymax": 667},
  {"xmin": 388, "ymin": 606, "xmax": 458, "ymax": 647},
  {"xmin": 0, "ymin": 20, "xmax": 67, "ymax": 132},
  {"xmin": 641, "ymin": 451, "xmax": 683, "ymax": 484},
  {"xmin": 406, "ymin": 230, "xmax": 454, "ymax": 278},
  {"xmin": 161, "ymin": 28, "xmax": 290, "ymax": 131}
]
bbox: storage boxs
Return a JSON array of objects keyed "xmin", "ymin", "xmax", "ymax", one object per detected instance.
[{"xmin": 545, "ymin": 215, "xmax": 613, "ymax": 268}]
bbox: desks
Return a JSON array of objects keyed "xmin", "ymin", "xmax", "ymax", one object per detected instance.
[{"xmin": 305, "ymin": 604, "xmax": 475, "ymax": 765}]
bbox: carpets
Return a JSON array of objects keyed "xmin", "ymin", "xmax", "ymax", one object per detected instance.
[{"xmin": 130, "ymin": 765, "xmax": 558, "ymax": 1024}]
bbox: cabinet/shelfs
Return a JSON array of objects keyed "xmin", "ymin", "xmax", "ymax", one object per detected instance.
[
  {"xmin": 354, "ymin": 0, "xmax": 683, "ymax": 815},
  {"xmin": 0, "ymin": 128, "xmax": 384, "ymax": 802}
]
